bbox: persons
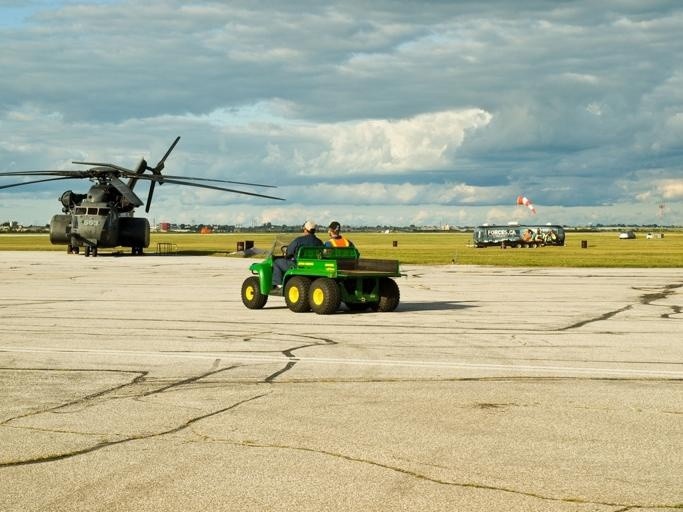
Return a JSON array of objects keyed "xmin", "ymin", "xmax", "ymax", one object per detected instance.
[
  {"xmin": 270, "ymin": 221, "xmax": 322, "ymax": 290},
  {"xmin": 521, "ymin": 229, "xmax": 568, "ymax": 250},
  {"xmin": 325, "ymin": 219, "xmax": 359, "ymax": 258}
]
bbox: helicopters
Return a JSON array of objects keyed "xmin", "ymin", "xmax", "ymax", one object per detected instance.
[{"xmin": 0, "ymin": 136, "xmax": 286, "ymax": 257}]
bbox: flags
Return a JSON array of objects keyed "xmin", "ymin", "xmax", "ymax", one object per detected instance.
[{"xmin": 517, "ymin": 193, "xmax": 536, "ymax": 214}]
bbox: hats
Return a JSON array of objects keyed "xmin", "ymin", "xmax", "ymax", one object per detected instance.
[
  {"xmin": 328, "ymin": 222, "xmax": 340, "ymax": 231},
  {"xmin": 305, "ymin": 220, "xmax": 316, "ymax": 232}
]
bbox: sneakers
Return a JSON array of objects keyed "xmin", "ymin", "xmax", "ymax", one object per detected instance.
[{"xmin": 269, "ymin": 287, "xmax": 282, "ymax": 294}]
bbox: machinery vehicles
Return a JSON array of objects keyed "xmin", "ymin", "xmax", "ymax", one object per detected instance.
[{"xmin": 241, "ymin": 238, "xmax": 403, "ymax": 314}]
